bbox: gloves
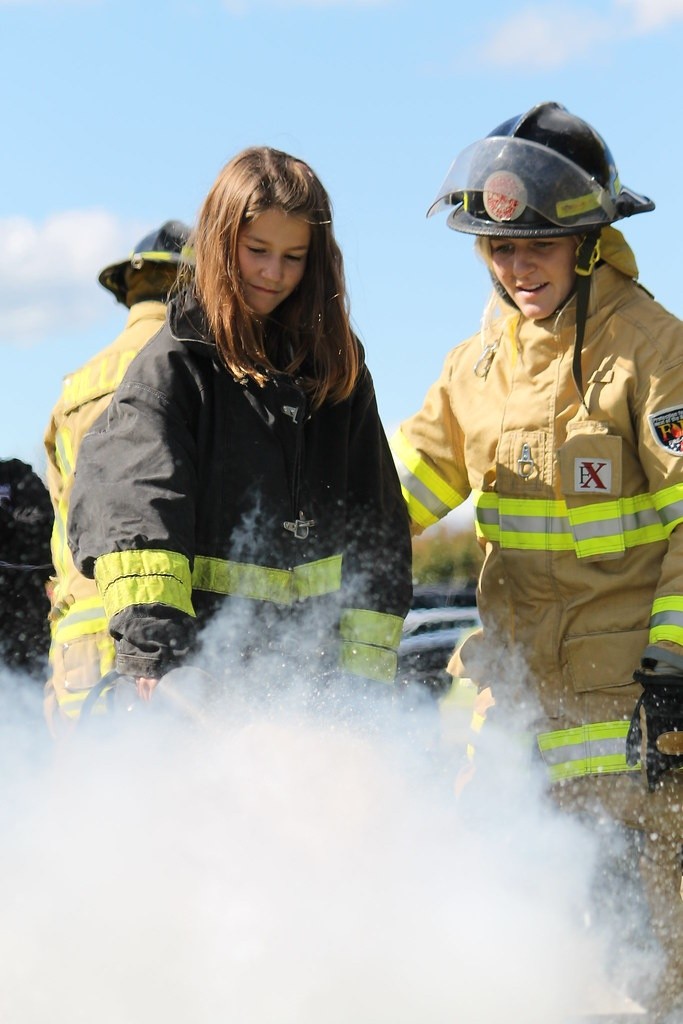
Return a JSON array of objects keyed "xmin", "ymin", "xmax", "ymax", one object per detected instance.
[{"xmin": 623, "ymin": 667, "xmax": 683, "ymax": 793}]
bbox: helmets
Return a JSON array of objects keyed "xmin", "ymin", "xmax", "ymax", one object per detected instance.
[
  {"xmin": 98, "ymin": 219, "xmax": 208, "ymax": 302},
  {"xmin": 425, "ymin": 99, "xmax": 656, "ymax": 235}
]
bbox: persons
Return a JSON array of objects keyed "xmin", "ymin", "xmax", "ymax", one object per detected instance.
[
  {"xmin": 63, "ymin": 142, "xmax": 415, "ymax": 748},
  {"xmin": 42, "ymin": 218, "xmax": 215, "ymax": 746},
  {"xmin": 384, "ymin": 100, "xmax": 683, "ymax": 1023},
  {"xmin": 0, "ymin": 454, "xmax": 56, "ymax": 686}
]
